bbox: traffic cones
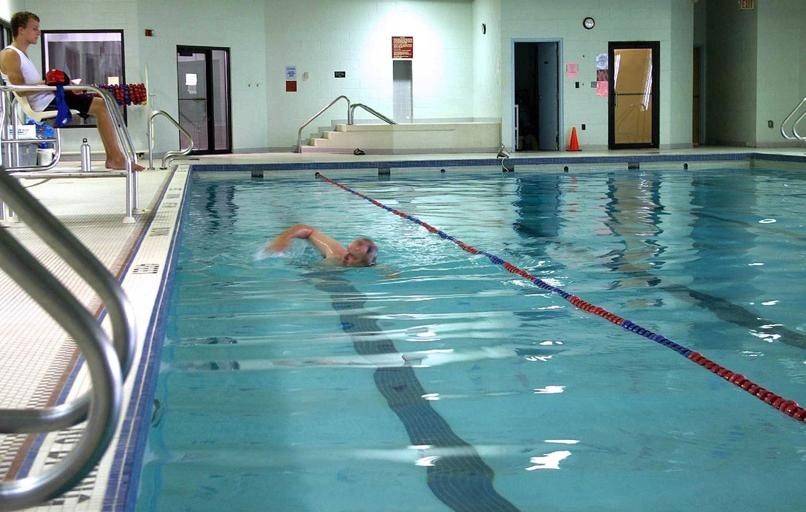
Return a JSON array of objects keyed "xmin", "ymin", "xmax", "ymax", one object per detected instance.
[{"xmin": 565, "ymin": 127, "xmax": 582, "ymax": 151}]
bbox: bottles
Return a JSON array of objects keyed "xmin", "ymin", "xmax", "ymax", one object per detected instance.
[{"xmin": 81, "ymin": 137, "xmax": 91, "ymax": 172}]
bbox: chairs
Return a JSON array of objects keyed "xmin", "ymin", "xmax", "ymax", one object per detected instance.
[{"xmin": 5, "ymin": 84, "xmax": 80, "ymax": 172}]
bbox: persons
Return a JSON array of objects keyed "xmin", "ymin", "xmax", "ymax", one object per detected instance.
[
  {"xmin": 0, "ymin": 11, "xmax": 146, "ymax": 172},
  {"xmin": 269, "ymin": 222, "xmax": 378, "ymax": 266}
]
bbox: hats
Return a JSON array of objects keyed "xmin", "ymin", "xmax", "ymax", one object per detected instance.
[{"xmin": 45, "ymin": 69, "xmax": 70, "ymax": 86}]
